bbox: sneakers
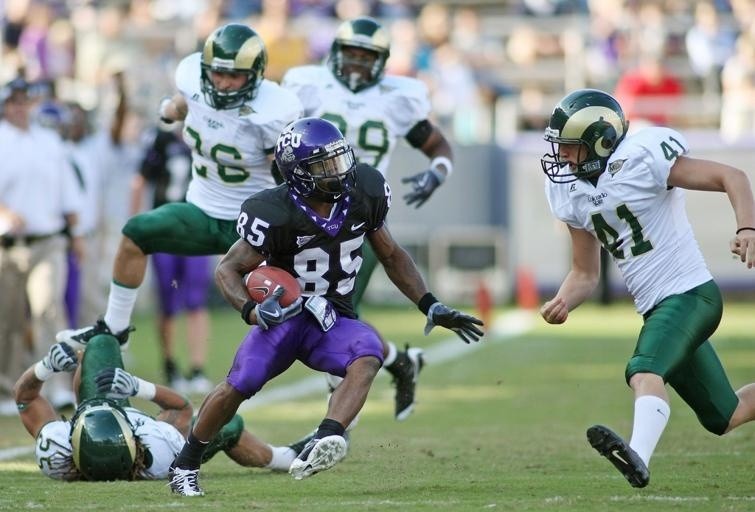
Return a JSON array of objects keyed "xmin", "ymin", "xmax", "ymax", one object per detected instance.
[
  {"xmin": 391, "ymin": 343, "xmax": 424, "ymax": 421},
  {"xmin": 587, "ymin": 425, "xmax": 650, "ymax": 487},
  {"xmin": 168, "ymin": 460, "xmax": 204, "ymax": 496},
  {"xmin": 55, "ymin": 317, "xmax": 135, "ymax": 351},
  {"xmin": 288, "ymin": 435, "xmax": 348, "ymax": 480},
  {"xmin": 288, "ymin": 427, "xmax": 318, "ymax": 456}
]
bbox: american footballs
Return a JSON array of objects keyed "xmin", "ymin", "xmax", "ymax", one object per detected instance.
[{"xmin": 242, "ymin": 265, "xmax": 302, "ymax": 306}]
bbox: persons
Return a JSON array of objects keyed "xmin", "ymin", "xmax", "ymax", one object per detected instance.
[
  {"xmin": 11, "ymin": 334, "xmax": 351, "ymax": 482},
  {"xmin": 539, "ymin": 90, "xmax": 755, "ymax": 489},
  {"xmin": 1, "ymin": 0, "xmax": 753, "ymax": 429},
  {"xmin": 167, "ymin": 118, "xmax": 485, "ymax": 499}
]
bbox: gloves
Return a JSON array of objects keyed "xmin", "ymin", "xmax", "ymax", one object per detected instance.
[
  {"xmin": 424, "ymin": 302, "xmax": 484, "ymax": 344},
  {"xmin": 43, "ymin": 341, "xmax": 78, "ymax": 372},
  {"xmin": 402, "ymin": 168, "xmax": 443, "ymax": 208},
  {"xmin": 255, "ymin": 285, "xmax": 303, "ymax": 331},
  {"xmin": 95, "ymin": 367, "xmax": 139, "ymax": 397}
]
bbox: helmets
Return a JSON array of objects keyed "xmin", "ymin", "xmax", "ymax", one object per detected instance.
[
  {"xmin": 200, "ymin": 24, "xmax": 266, "ymax": 110},
  {"xmin": 275, "ymin": 117, "xmax": 357, "ymax": 203},
  {"xmin": 540, "ymin": 88, "xmax": 626, "ymax": 183},
  {"xmin": 330, "ymin": 18, "xmax": 390, "ymax": 92},
  {"xmin": 69, "ymin": 398, "xmax": 136, "ymax": 480}
]
